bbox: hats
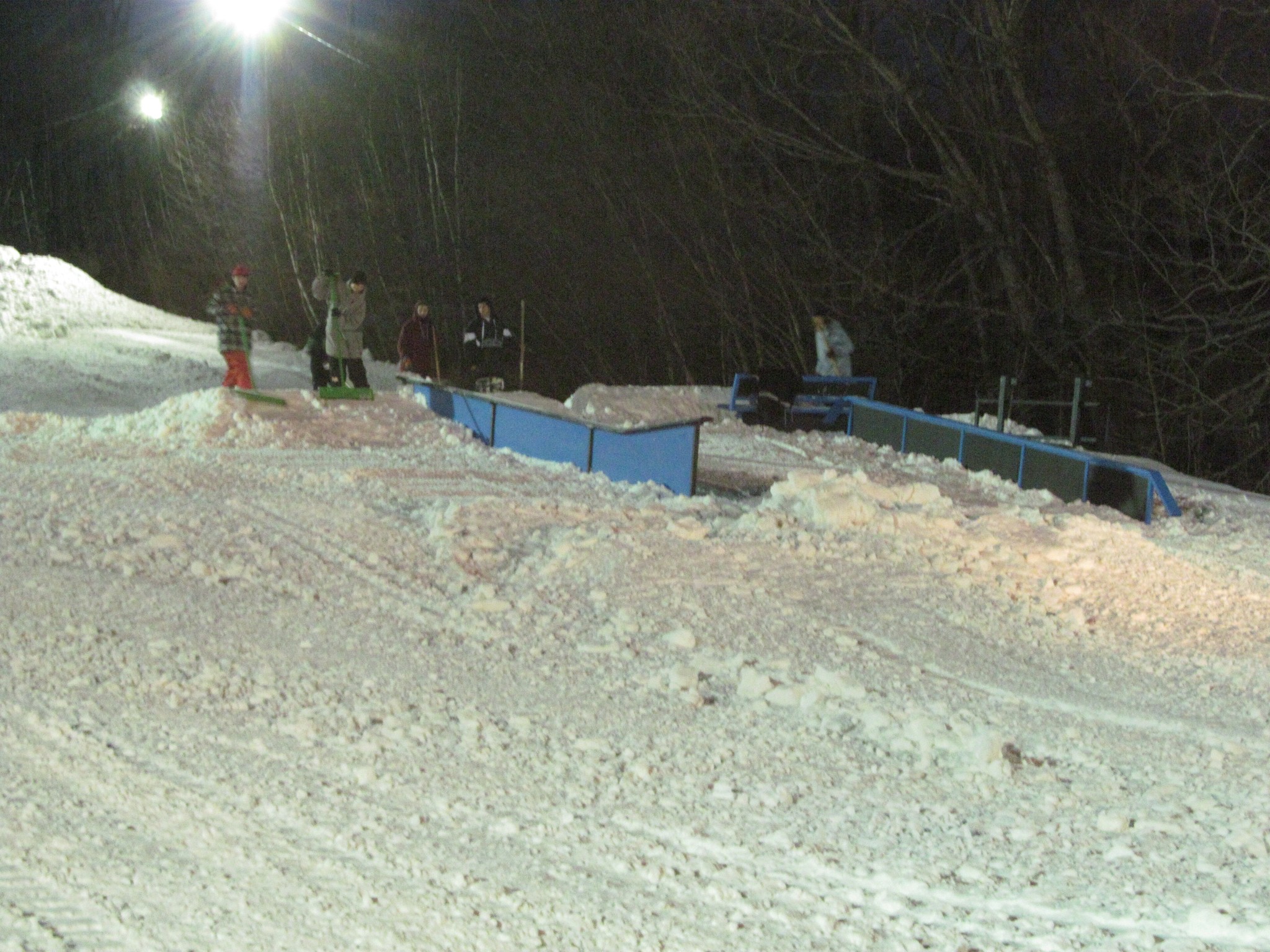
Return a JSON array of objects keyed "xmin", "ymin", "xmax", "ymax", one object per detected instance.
[
  {"xmin": 351, "ymin": 271, "xmax": 367, "ymax": 284},
  {"xmin": 232, "ymin": 266, "xmax": 248, "ymax": 276}
]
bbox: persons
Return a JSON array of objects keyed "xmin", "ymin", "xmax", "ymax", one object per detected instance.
[
  {"xmin": 306, "ymin": 317, "xmax": 329, "ymax": 390},
  {"xmin": 205, "ymin": 265, "xmax": 258, "ymax": 389},
  {"xmin": 311, "ymin": 269, "xmax": 370, "ymax": 387},
  {"xmin": 397, "ymin": 300, "xmax": 446, "ymax": 378},
  {"xmin": 463, "ymin": 299, "xmax": 512, "ymax": 392},
  {"xmin": 811, "ymin": 306, "xmax": 854, "ymax": 394}
]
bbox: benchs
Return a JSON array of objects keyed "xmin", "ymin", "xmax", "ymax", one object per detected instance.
[{"xmin": 720, "ymin": 371, "xmax": 878, "ymax": 430}]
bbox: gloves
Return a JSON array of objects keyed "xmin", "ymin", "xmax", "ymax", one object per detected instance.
[
  {"xmin": 229, "ymin": 305, "xmax": 237, "ymax": 314},
  {"xmin": 332, "ymin": 308, "xmax": 341, "ymax": 318},
  {"xmin": 241, "ymin": 308, "xmax": 253, "ymax": 318},
  {"xmin": 324, "ymin": 269, "xmax": 334, "ymax": 277}
]
